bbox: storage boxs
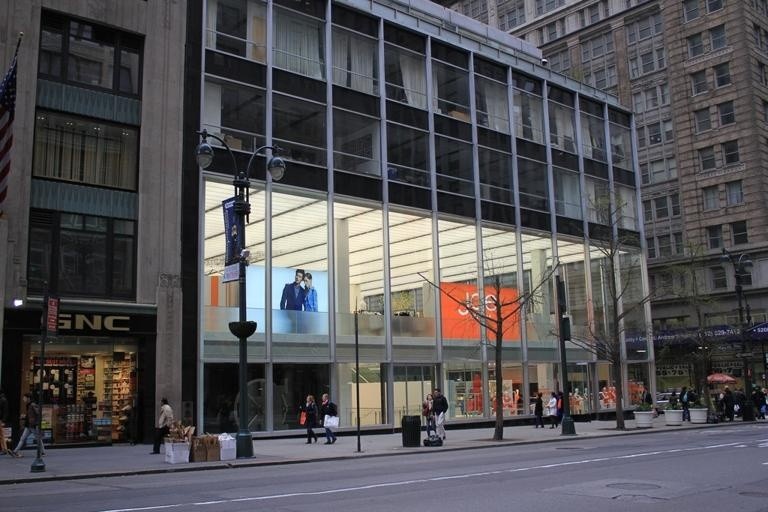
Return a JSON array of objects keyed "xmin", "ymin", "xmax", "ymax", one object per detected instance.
[{"xmin": 164, "ymin": 432, "xmax": 237, "ymax": 465}]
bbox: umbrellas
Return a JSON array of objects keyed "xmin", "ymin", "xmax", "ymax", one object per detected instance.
[{"xmin": 706, "ymin": 372, "xmax": 736, "ymax": 384}]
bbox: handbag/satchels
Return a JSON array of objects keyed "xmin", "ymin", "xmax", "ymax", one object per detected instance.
[
  {"xmin": 328, "ymin": 401, "xmax": 338, "ymax": 416},
  {"xmin": 300, "ymin": 409, "xmax": 308, "ymax": 426},
  {"xmin": 422, "ymin": 400, "xmax": 429, "ymax": 416}
]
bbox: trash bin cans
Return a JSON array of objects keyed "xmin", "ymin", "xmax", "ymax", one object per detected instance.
[
  {"xmin": 743, "ymin": 401, "xmax": 754, "ymax": 420},
  {"xmin": 402, "ymin": 415, "xmax": 420, "ymax": 447}
]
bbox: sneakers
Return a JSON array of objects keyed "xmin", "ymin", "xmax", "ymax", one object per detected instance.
[
  {"xmin": 7, "ymin": 449, "xmax": 24, "ymax": 458},
  {"xmin": 439, "ymin": 436, "xmax": 447, "ymax": 441},
  {"xmin": 535, "ymin": 425, "xmax": 554, "ymax": 429},
  {"xmin": 148, "ymin": 450, "xmax": 161, "ymax": 455},
  {"xmin": 41, "ymin": 453, "xmax": 49, "ymax": 457},
  {"xmin": 0, "ymin": 451, "xmax": 7, "ymax": 455},
  {"xmin": 305, "ymin": 436, "xmax": 318, "ymax": 445},
  {"xmin": 324, "ymin": 437, "xmax": 338, "ymax": 445}
]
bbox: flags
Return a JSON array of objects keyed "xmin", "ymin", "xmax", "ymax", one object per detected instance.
[{"xmin": 0, "ymin": 56, "xmax": 17, "ymax": 228}]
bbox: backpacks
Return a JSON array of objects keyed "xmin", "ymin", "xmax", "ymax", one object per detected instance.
[
  {"xmin": 682, "ymin": 391, "xmax": 688, "ymax": 403},
  {"xmin": 322, "ymin": 414, "xmax": 339, "ymax": 430},
  {"xmin": 311, "ymin": 403, "xmax": 318, "ymax": 417}
]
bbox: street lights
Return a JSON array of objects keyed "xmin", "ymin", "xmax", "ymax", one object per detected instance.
[
  {"xmin": 196, "ymin": 129, "xmax": 286, "ymax": 458},
  {"xmin": 722, "ymin": 248, "xmax": 756, "ymax": 420}
]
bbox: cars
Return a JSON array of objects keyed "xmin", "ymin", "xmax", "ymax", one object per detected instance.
[{"xmin": 656, "ymin": 393, "xmax": 680, "ymax": 410}]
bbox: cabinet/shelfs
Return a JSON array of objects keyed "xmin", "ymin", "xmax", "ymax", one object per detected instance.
[{"xmin": 30, "ymin": 353, "xmax": 138, "ymax": 441}]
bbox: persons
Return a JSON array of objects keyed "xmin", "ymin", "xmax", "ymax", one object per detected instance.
[
  {"xmin": 319, "ymin": 393, "xmax": 337, "ymax": 444},
  {"xmin": 488, "ymin": 386, "xmax": 521, "ymax": 417},
  {"xmin": 430, "ymin": 387, "xmax": 449, "ymax": 440},
  {"xmin": 640, "ymin": 385, "xmax": 652, "ymax": 406},
  {"xmin": 8, "ymin": 394, "xmax": 47, "ymax": 457},
  {"xmin": 544, "ymin": 391, "xmax": 558, "ymax": 429},
  {"xmin": 533, "ymin": 392, "xmax": 545, "ymax": 428},
  {"xmin": 279, "ymin": 269, "xmax": 305, "ymax": 311},
  {"xmin": 148, "ymin": 396, "xmax": 175, "ymax": 454},
  {"xmin": 302, "ymin": 273, "xmax": 318, "ymax": 311},
  {"xmin": 556, "ymin": 391, "xmax": 564, "ymax": 424},
  {"xmin": 568, "ymin": 383, "xmax": 616, "ymax": 415},
  {"xmin": 653, "ymin": 384, "xmax": 768, "ymax": 425},
  {"xmin": 298, "ymin": 395, "xmax": 318, "ymax": 444},
  {"xmin": 0, "ymin": 391, "xmax": 9, "ymax": 455},
  {"xmin": 422, "ymin": 394, "xmax": 436, "ymax": 437}
]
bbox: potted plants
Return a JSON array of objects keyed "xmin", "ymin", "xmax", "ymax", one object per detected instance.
[
  {"xmin": 688, "ymin": 384, "xmax": 709, "ymax": 424},
  {"xmin": 663, "ymin": 396, "xmax": 685, "ymax": 426},
  {"xmin": 633, "ymin": 383, "xmax": 655, "ymax": 428}
]
